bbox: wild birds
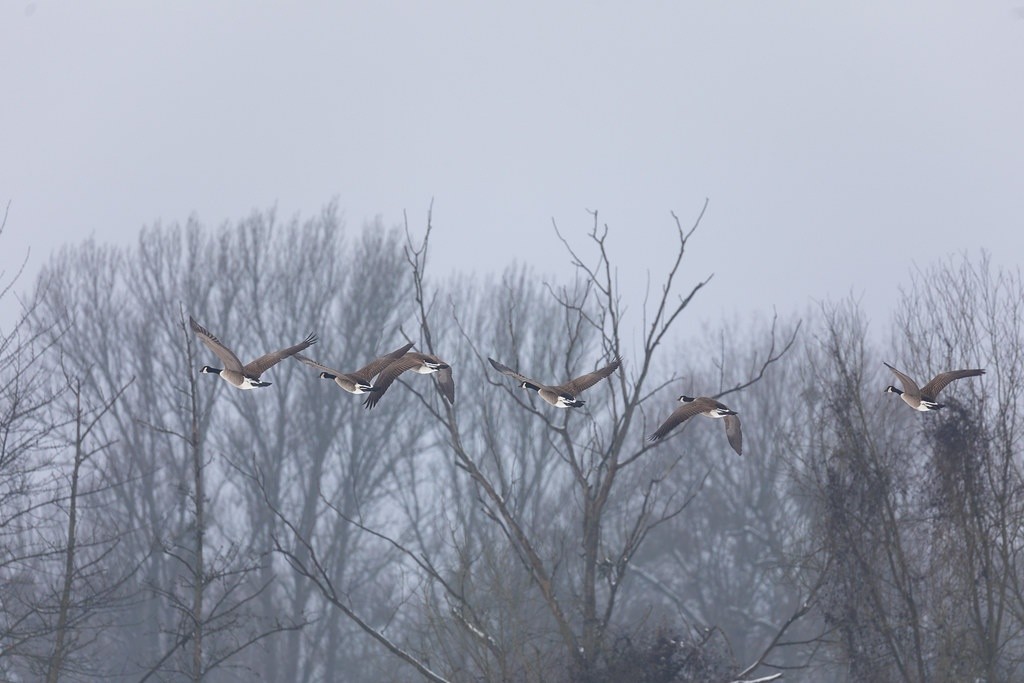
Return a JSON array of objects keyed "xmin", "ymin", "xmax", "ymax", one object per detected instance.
[
  {"xmin": 487, "ymin": 355, "xmax": 624, "ymax": 409},
  {"xmin": 361, "ymin": 352, "xmax": 455, "ymax": 411},
  {"xmin": 275, "ymin": 341, "xmax": 419, "ymax": 395},
  {"xmin": 188, "ymin": 315, "xmax": 320, "ymax": 391},
  {"xmin": 646, "ymin": 395, "xmax": 743, "ymax": 456},
  {"xmin": 881, "ymin": 360, "xmax": 987, "ymax": 412}
]
